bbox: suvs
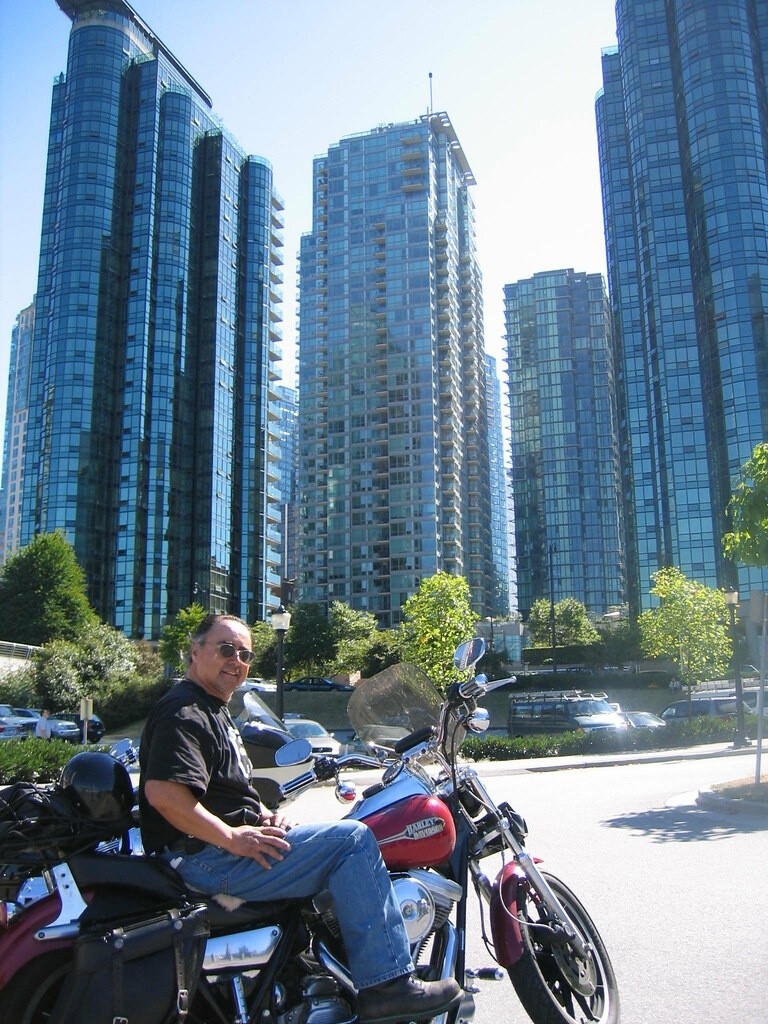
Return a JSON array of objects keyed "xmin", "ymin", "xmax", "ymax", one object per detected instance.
[
  {"xmin": 25, "ymin": 708, "xmax": 81, "ymax": 740},
  {"xmin": 659, "ymin": 695, "xmax": 753, "ymax": 726},
  {"xmin": 54, "ymin": 712, "xmax": 106, "ymax": 742},
  {"xmin": 0, "ymin": 704, "xmax": 27, "ymax": 742},
  {"xmin": 14, "ymin": 708, "xmax": 41, "ymax": 739}
]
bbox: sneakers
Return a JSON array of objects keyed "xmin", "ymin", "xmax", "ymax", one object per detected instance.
[{"xmin": 357, "ymin": 972, "xmax": 466, "ymax": 1023}]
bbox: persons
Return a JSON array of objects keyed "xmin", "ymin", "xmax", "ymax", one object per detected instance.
[
  {"xmin": 36, "ymin": 709, "xmax": 51, "ymax": 743},
  {"xmin": 138, "ymin": 615, "xmax": 464, "ymax": 1024},
  {"xmin": 669, "ymin": 678, "xmax": 681, "ymax": 693}
]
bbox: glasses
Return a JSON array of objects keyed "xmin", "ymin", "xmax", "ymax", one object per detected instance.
[{"xmin": 199, "ymin": 641, "xmax": 255, "ymax": 664}]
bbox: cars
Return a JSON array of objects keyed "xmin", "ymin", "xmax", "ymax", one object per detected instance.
[
  {"xmin": 618, "ymin": 710, "xmax": 667, "ymax": 730},
  {"xmin": 243, "ymin": 678, "xmax": 277, "ymax": 693},
  {"xmin": 281, "ymin": 718, "xmax": 343, "ymax": 759},
  {"xmin": 283, "ymin": 674, "xmax": 357, "ymax": 692},
  {"xmin": 338, "ymin": 724, "xmax": 431, "ymax": 764}
]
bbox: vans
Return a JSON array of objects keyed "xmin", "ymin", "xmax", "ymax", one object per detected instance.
[
  {"xmin": 506, "ymin": 689, "xmax": 628, "ymax": 740},
  {"xmin": 730, "ymin": 686, "xmax": 768, "ymax": 721}
]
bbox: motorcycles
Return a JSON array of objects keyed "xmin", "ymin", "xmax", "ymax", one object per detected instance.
[{"xmin": 0, "ymin": 638, "xmax": 623, "ymax": 1024}]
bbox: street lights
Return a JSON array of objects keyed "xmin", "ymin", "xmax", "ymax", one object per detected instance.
[{"xmin": 269, "ymin": 604, "xmax": 292, "ymax": 726}]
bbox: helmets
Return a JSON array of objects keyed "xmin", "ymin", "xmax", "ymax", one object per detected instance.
[{"xmin": 60, "ymin": 752, "xmax": 134, "ymax": 823}]
